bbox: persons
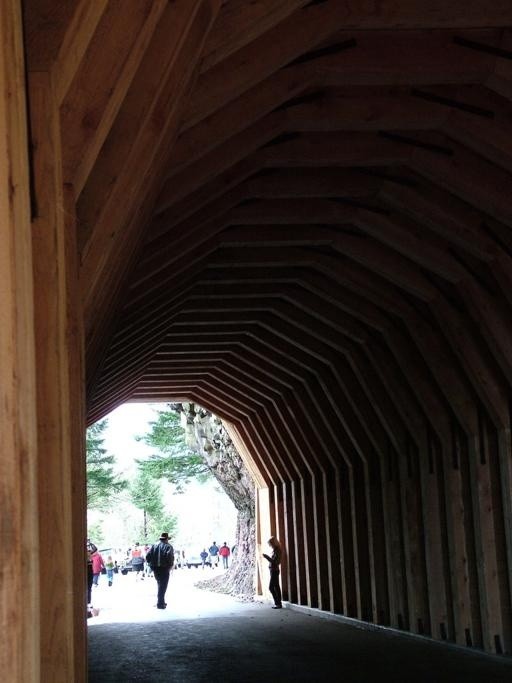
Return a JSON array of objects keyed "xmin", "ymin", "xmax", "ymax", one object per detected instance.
[
  {"xmin": 200, "ymin": 548, "xmax": 208, "ymax": 568},
  {"xmin": 173, "ymin": 551, "xmax": 187, "ymax": 571},
  {"xmin": 87, "ymin": 552, "xmax": 93, "ymax": 608},
  {"xmin": 209, "ymin": 541, "xmax": 219, "ymax": 563},
  {"xmin": 263, "ymin": 536, "xmax": 282, "ymax": 609},
  {"xmin": 90, "ymin": 551, "xmax": 104, "ymax": 586},
  {"xmin": 124, "ymin": 542, "xmax": 154, "ymax": 581},
  {"xmin": 145, "ymin": 532, "xmax": 174, "ymax": 609},
  {"xmin": 219, "ymin": 542, "xmax": 230, "ymax": 569},
  {"xmin": 104, "ymin": 556, "xmax": 116, "ymax": 586}
]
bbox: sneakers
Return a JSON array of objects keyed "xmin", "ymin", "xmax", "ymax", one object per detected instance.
[{"xmin": 272, "ymin": 604, "xmax": 282, "ymax": 609}]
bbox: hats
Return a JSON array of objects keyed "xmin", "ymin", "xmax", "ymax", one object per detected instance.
[{"xmin": 160, "ymin": 533, "xmax": 171, "ymax": 540}]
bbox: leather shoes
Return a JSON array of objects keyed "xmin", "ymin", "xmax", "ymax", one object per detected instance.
[{"xmin": 157, "ymin": 603, "xmax": 167, "ymax": 609}]
